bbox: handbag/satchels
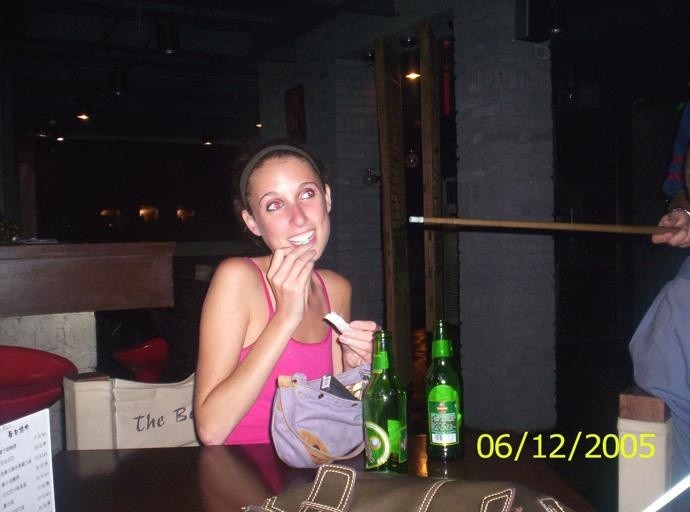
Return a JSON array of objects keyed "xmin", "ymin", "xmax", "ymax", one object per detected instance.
[
  {"xmin": 269, "ymin": 362, "xmax": 371, "ymax": 472},
  {"xmin": 239, "ymin": 464, "xmax": 576, "ymax": 512}
]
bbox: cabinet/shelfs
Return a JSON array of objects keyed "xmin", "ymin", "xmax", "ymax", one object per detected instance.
[{"xmin": 15, "ymin": 35, "xmax": 262, "ymax": 256}]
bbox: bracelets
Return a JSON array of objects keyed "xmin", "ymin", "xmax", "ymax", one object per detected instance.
[{"xmin": 671, "ymin": 206, "xmax": 690, "ymax": 217}]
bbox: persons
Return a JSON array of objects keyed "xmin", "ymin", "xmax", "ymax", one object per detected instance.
[
  {"xmin": 650, "ymin": 103, "xmax": 690, "ymax": 249},
  {"xmin": 628, "ymin": 145, "xmax": 690, "ymax": 512},
  {"xmin": 192, "ymin": 137, "xmax": 386, "ymax": 446},
  {"xmin": 196, "ymin": 445, "xmax": 290, "ymax": 512}
]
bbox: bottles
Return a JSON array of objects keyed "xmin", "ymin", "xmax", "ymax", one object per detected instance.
[
  {"xmin": 423, "ymin": 318, "xmax": 464, "ymax": 463},
  {"xmin": 361, "ymin": 328, "xmax": 409, "ymax": 475}
]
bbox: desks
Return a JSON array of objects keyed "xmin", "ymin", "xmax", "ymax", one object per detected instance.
[{"xmin": 54, "ymin": 428, "xmax": 595, "ymax": 512}]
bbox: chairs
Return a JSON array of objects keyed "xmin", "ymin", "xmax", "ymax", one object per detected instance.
[{"xmin": 63, "ymin": 369, "xmax": 202, "ymax": 450}]
